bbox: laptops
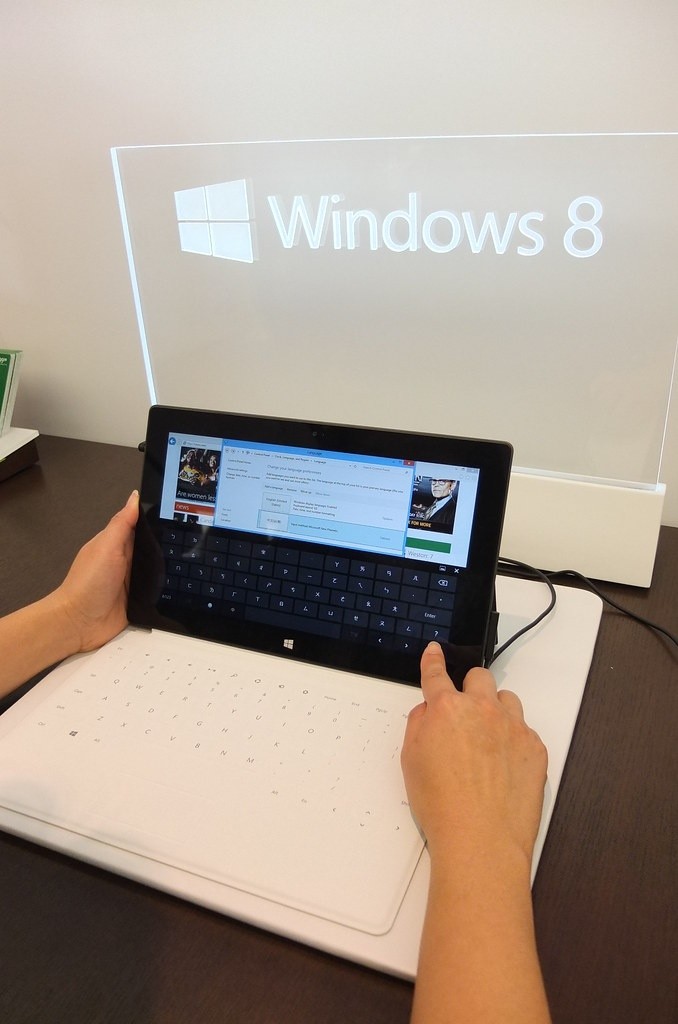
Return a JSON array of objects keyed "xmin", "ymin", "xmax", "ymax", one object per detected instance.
[{"xmin": 0, "ymin": 403, "xmax": 513, "ymax": 937}]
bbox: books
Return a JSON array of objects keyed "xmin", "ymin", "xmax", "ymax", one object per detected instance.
[{"xmin": 0, "ymin": 352, "xmax": 21, "ymax": 430}]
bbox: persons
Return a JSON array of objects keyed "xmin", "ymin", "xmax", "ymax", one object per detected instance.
[
  {"xmin": 0, "ymin": 490, "xmax": 559, "ymax": 1024},
  {"xmin": 422, "ymin": 477, "xmax": 458, "ymax": 524},
  {"xmin": 180, "ymin": 449, "xmax": 220, "ymax": 481}
]
